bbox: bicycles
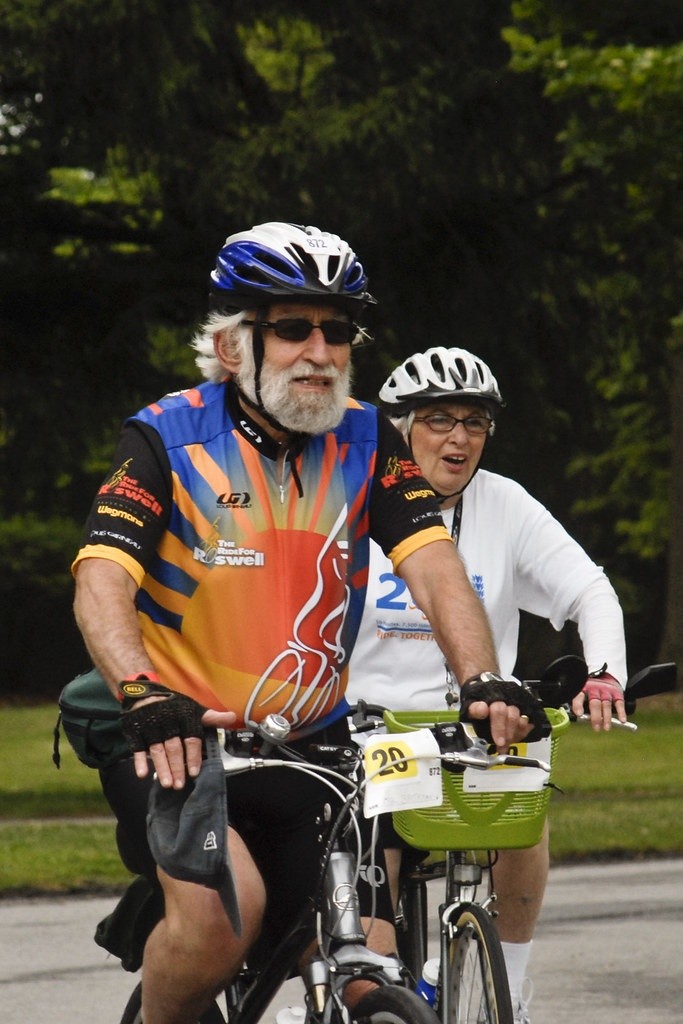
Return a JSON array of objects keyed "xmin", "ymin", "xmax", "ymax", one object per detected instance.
[
  {"xmin": 105, "ymin": 700, "xmax": 556, "ymax": 1024},
  {"xmin": 303, "ymin": 659, "xmax": 679, "ymax": 1024}
]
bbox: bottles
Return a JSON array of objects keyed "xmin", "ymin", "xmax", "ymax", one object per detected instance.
[
  {"xmin": 415, "ymin": 956, "xmax": 441, "ymax": 1013},
  {"xmin": 274, "ymin": 1007, "xmax": 307, "ymax": 1024}
]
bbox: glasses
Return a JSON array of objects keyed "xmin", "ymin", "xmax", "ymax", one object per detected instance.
[
  {"xmin": 236, "ymin": 317, "xmax": 361, "ymax": 345},
  {"xmin": 413, "ymin": 413, "xmax": 492, "ymax": 436}
]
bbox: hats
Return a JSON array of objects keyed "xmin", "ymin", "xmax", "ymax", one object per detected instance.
[{"xmin": 146, "ymin": 728, "xmax": 242, "ymax": 934}]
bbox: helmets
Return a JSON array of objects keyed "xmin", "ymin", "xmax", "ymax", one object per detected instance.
[
  {"xmin": 374, "ymin": 344, "xmax": 505, "ymax": 421},
  {"xmin": 208, "ymin": 221, "xmax": 378, "ymax": 318}
]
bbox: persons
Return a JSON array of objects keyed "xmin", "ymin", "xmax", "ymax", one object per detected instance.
[
  {"xmin": 328, "ymin": 344, "xmax": 629, "ymax": 1024},
  {"xmin": 71, "ymin": 221, "xmax": 547, "ymax": 1024}
]
